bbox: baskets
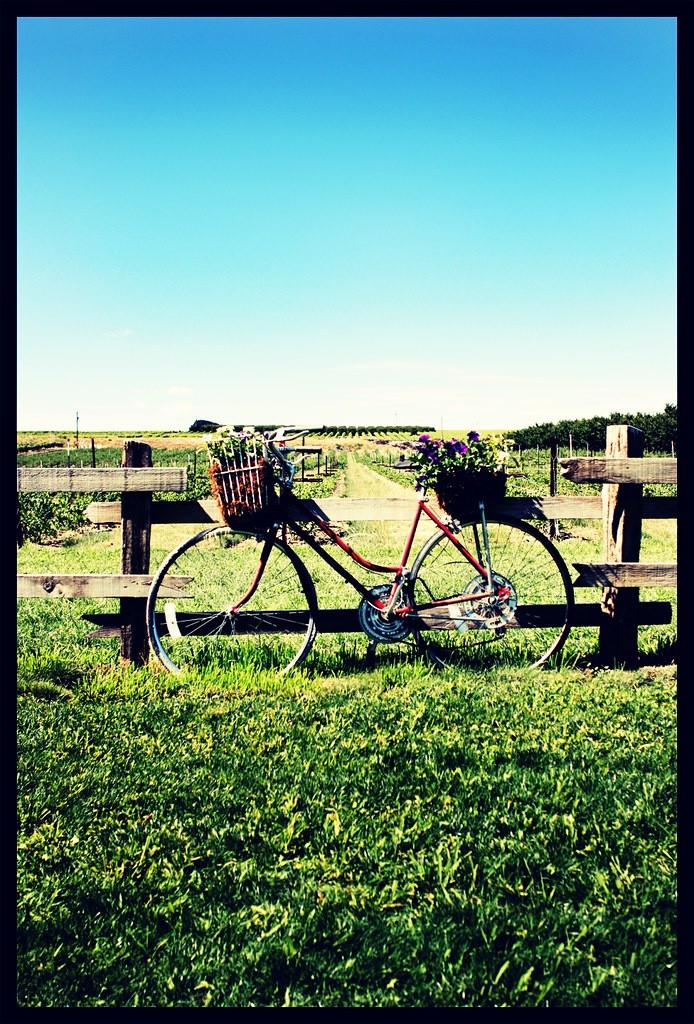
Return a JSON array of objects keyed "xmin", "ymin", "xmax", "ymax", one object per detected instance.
[{"xmin": 206, "ymin": 436, "xmax": 275, "ymax": 530}]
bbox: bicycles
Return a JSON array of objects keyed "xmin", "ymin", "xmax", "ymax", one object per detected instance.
[{"xmin": 139, "ymin": 421, "xmax": 574, "ymax": 686}]
